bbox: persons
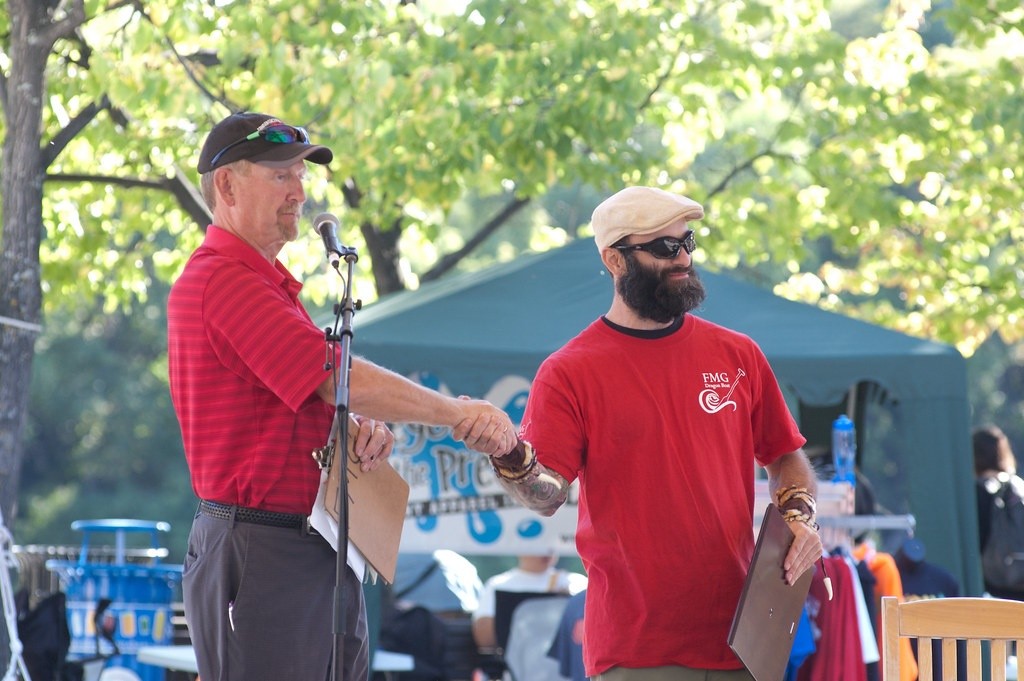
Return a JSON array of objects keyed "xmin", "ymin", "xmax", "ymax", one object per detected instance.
[
  {"xmin": 167, "ymin": 112, "xmax": 516, "ymax": 681},
  {"xmin": 449, "ymin": 181, "xmax": 835, "ymax": 681},
  {"xmin": 971, "ymin": 425, "xmax": 1024, "ymax": 601}
]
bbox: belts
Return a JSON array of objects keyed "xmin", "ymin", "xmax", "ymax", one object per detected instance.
[{"xmin": 200, "ymin": 499, "xmax": 320, "ymax": 536}]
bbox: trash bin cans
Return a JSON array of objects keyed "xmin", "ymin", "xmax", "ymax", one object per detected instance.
[{"xmin": 45, "ymin": 519, "xmax": 184, "ymax": 681}]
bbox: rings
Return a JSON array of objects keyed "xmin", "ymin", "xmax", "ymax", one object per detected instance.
[{"xmin": 503, "ymin": 426, "xmax": 508, "ymax": 432}]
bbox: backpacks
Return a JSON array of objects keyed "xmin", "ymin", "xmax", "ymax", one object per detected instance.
[{"xmin": 982, "ymin": 472, "xmax": 1024, "ymax": 597}]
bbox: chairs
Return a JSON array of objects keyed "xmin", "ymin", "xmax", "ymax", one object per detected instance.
[{"xmin": 881, "ymin": 596, "xmax": 1024, "ymax": 681}]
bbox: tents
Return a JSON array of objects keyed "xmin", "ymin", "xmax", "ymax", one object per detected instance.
[{"xmin": 317, "ymin": 235, "xmax": 1024, "ymax": 598}]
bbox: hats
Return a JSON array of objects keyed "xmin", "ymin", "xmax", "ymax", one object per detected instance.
[
  {"xmin": 197, "ymin": 112, "xmax": 334, "ymax": 175},
  {"xmin": 591, "ymin": 186, "xmax": 705, "ymax": 257}
]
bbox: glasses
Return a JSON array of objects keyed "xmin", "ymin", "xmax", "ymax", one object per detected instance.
[
  {"xmin": 208, "ymin": 125, "xmax": 310, "ymax": 167},
  {"xmin": 614, "ymin": 228, "xmax": 696, "ymax": 259}
]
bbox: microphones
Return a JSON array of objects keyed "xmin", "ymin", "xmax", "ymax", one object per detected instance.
[{"xmin": 314, "ymin": 212, "xmax": 341, "ymax": 268}]
